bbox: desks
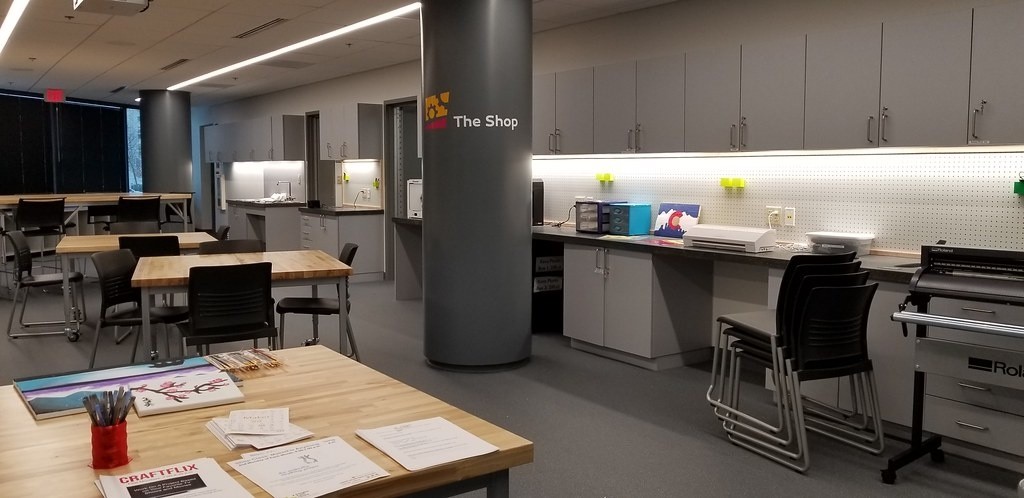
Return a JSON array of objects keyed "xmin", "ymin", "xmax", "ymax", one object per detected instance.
[
  {"xmin": 391, "ymin": 218, "xmax": 424, "ymax": 302},
  {"xmin": 226, "ymin": 200, "xmax": 307, "ymax": 252},
  {"xmin": 132, "ymin": 250, "xmax": 352, "ymax": 357},
  {"xmin": 1, "ymin": 193, "xmax": 193, "ymax": 265},
  {"xmin": 0, "ymin": 343, "xmax": 534, "ymax": 498},
  {"xmin": 531, "ymin": 225, "xmax": 1023, "ymax": 305},
  {"xmin": 56, "ymin": 232, "xmax": 219, "ymax": 343}
]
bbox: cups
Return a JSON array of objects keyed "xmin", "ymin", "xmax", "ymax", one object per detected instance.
[{"xmin": 91, "ymin": 418, "xmax": 129, "ymax": 469}]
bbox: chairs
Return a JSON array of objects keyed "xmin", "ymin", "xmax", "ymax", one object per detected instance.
[
  {"xmin": 706, "ymin": 252, "xmax": 881, "ymax": 475},
  {"xmin": 0, "ymin": 191, "xmax": 361, "ymax": 365}
]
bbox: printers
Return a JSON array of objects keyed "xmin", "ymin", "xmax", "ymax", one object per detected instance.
[{"xmin": 682, "ymin": 223, "xmax": 777, "ymax": 253}]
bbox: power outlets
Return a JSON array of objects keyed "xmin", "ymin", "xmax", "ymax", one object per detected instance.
[
  {"xmin": 764, "ymin": 206, "xmax": 795, "ymax": 227},
  {"xmin": 362, "ymin": 188, "xmax": 370, "ymax": 199}
]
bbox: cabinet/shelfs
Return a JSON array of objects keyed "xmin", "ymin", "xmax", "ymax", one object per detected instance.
[
  {"xmin": 563, "ymin": 242, "xmax": 714, "ymax": 373},
  {"xmin": 300, "ymin": 209, "xmax": 384, "ymax": 282},
  {"xmin": 406, "ymin": 179, "xmax": 422, "ymax": 220},
  {"xmin": 320, "ymin": 103, "xmax": 382, "ymax": 161},
  {"xmin": 204, "ymin": 114, "xmax": 305, "ymax": 163},
  {"xmin": 765, "ymin": 265, "xmax": 1024, "ymax": 482},
  {"xmin": 531, "ymin": 4, "xmax": 1024, "ymax": 157}
]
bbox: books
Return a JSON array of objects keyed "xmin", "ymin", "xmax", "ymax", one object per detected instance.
[
  {"xmin": 653, "ymin": 202, "xmax": 701, "ymax": 239},
  {"xmin": 128, "ymin": 371, "xmax": 245, "ymax": 417}
]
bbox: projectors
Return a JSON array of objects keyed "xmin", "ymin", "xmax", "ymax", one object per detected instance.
[{"xmin": 73, "ymin": 0, "xmax": 149, "ymax": 16}]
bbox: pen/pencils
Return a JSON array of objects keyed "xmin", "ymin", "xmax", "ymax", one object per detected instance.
[{"xmin": 82, "ymin": 386, "xmax": 136, "ymax": 426}]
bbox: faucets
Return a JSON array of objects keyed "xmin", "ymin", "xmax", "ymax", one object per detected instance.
[{"xmin": 276, "ymin": 181, "xmax": 296, "ymax": 202}]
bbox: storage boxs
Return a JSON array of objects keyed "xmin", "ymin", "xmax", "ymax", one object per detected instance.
[{"xmin": 805, "ymin": 231, "xmax": 876, "ymax": 256}]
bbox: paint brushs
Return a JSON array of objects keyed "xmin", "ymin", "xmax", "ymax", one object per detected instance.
[{"xmin": 208, "ymin": 347, "xmax": 284, "ymax": 373}]
